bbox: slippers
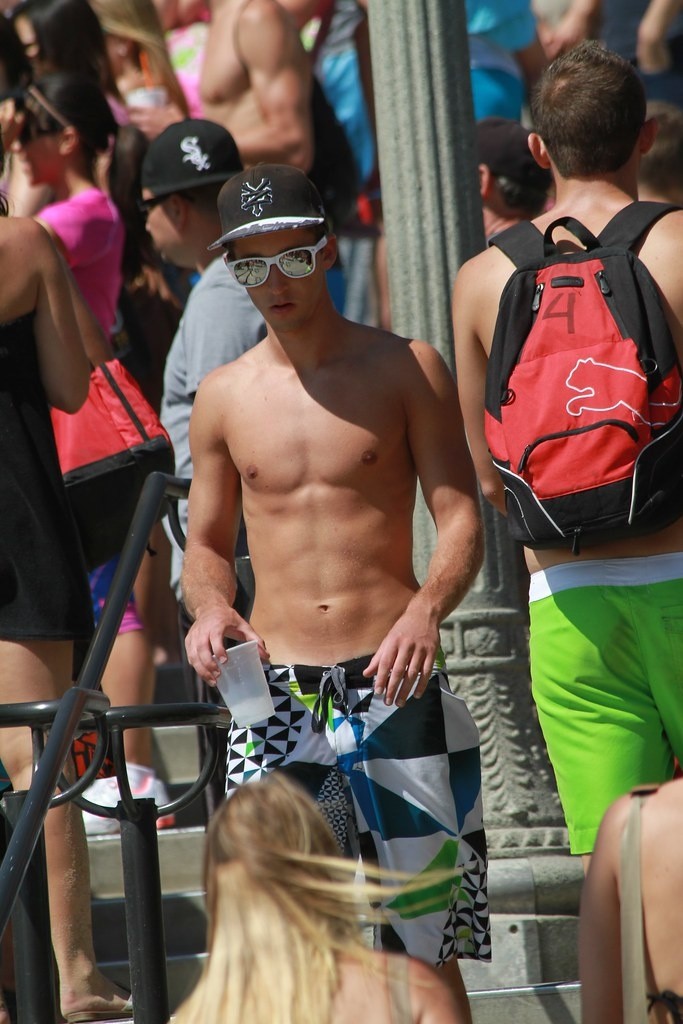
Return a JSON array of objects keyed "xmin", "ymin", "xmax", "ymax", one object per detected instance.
[{"xmin": 66, "ymin": 978, "xmax": 137, "ymax": 1024}]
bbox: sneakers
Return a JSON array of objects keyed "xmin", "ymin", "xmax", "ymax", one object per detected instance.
[
  {"xmin": 81, "ymin": 777, "xmax": 168, "ymax": 835},
  {"xmin": 145, "ymin": 777, "xmax": 174, "ymax": 829}
]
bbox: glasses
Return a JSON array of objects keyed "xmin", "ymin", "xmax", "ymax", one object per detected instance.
[{"xmin": 224, "ymin": 234, "xmax": 329, "ymax": 288}]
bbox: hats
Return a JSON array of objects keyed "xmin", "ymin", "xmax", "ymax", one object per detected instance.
[
  {"xmin": 207, "ymin": 160, "xmax": 326, "ymax": 251},
  {"xmin": 473, "ymin": 110, "xmax": 556, "ymax": 189},
  {"xmin": 134, "ymin": 119, "xmax": 244, "ymax": 199}
]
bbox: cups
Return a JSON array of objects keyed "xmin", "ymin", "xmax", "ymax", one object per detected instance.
[
  {"xmin": 125, "ymin": 86, "xmax": 166, "ymax": 111},
  {"xmin": 212, "ymin": 640, "xmax": 276, "ymax": 729}
]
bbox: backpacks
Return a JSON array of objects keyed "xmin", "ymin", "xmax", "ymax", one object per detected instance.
[{"xmin": 484, "ymin": 200, "xmax": 683, "ymax": 552}]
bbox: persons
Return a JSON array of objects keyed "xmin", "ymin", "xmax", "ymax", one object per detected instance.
[{"xmin": 0, "ymin": 0, "xmax": 683, "ymax": 1024}]
